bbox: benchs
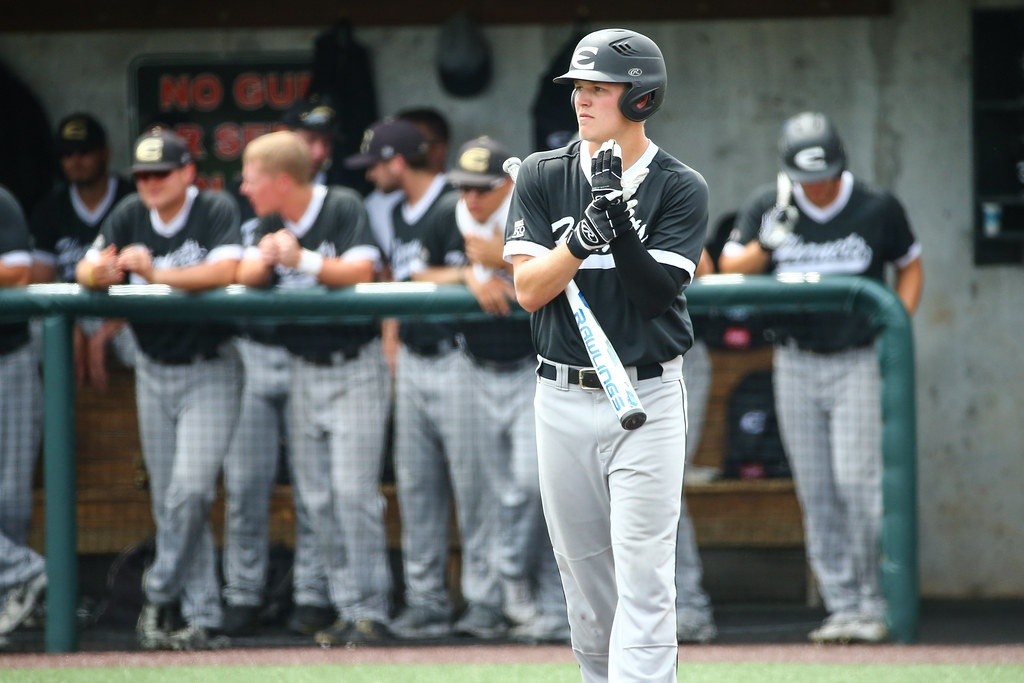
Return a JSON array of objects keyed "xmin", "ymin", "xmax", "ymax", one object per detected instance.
[{"xmin": 27, "ymin": 345, "xmax": 821, "ymax": 608}]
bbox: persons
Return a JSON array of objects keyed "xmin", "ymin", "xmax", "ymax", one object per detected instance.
[
  {"xmin": 720, "ymin": 109, "xmax": 926, "ymax": 643},
  {"xmin": 502, "ymin": 26, "xmax": 708, "ymax": 683},
  {"xmin": 0, "ymin": 104, "xmax": 719, "ymax": 649}
]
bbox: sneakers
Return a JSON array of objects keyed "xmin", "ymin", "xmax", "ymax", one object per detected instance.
[
  {"xmin": 0, "ymin": 567, "xmax": 48, "ymax": 634},
  {"xmin": 675, "ymin": 605, "xmax": 718, "ymax": 643}
]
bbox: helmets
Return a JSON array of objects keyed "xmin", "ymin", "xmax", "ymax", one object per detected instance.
[
  {"xmin": 552, "ymin": 28, "xmax": 668, "ymax": 123},
  {"xmin": 779, "ymin": 109, "xmax": 845, "ymax": 183}
]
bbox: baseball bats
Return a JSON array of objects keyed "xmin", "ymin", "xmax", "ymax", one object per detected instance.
[{"xmin": 561, "ymin": 277, "xmax": 647, "ymax": 431}]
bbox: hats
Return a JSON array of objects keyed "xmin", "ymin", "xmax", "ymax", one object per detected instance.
[
  {"xmin": 54, "ymin": 114, "xmax": 106, "ymax": 154},
  {"xmin": 282, "ymin": 101, "xmax": 341, "ymax": 135},
  {"xmin": 342, "ymin": 117, "xmax": 431, "ymax": 171},
  {"xmin": 445, "ymin": 133, "xmax": 517, "ymax": 188},
  {"xmin": 121, "ymin": 124, "xmax": 191, "ymax": 176}
]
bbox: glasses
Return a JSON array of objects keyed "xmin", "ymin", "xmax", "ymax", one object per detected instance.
[
  {"xmin": 135, "ymin": 169, "xmax": 174, "ymax": 182},
  {"xmin": 459, "ymin": 181, "xmax": 496, "ymax": 196}
]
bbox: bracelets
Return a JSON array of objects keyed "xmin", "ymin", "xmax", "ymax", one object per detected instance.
[
  {"xmin": 298, "ymin": 248, "xmax": 323, "ymax": 276},
  {"xmin": 88, "ymin": 266, "xmax": 95, "ymax": 287}
]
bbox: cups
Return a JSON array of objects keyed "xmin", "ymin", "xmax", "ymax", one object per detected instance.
[{"xmin": 981, "ymin": 202, "xmax": 1000, "ymax": 236}]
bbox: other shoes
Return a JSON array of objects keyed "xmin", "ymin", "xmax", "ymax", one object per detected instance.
[
  {"xmin": 709, "ymin": 458, "xmax": 757, "ymax": 484},
  {"xmin": 808, "ymin": 618, "xmax": 856, "ymax": 645},
  {"xmin": 839, "ymin": 617, "xmax": 888, "ymax": 644},
  {"xmin": 757, "ymin": 458, "xmax": 792, "ymax": 479},
  {"xmin": 137, "ymin": 603, "xmax": 569, "ymax": 649}
]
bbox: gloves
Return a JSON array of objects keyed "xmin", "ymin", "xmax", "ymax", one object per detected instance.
[
  {"xmin": 759, "ymin": 204, "xmax": 798, "ymax": 252},
  {"xmin": 574, "ymin": 190, "xmax": 638, "ymax": 253},
  {"xmin": 592, "ymin": 139, "xmax": 624, "ymax": 209}
]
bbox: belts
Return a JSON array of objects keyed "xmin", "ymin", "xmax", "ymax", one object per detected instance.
[
  {"xmin": 772, "ymin": 335, "xmax": 873, "ymax": 355},
  {"xmin": 141, "ymin": 346, "xmax": 221, "ymax": 366},
  {"xmin": 462, "ymin": 343, "xmax": 536, "ymax": 373},
  {"xmin": 287, "ymin": 344, "xmax": 359, "ymax": 366},
  {"xmin": 401, "ymin": 332, "xmax": 465, "ymax": 359},
  {"xmin": 232, "ymin": 327, "xmax": 286, "ymax": 347},
  {"xmin": 538, "ymin": 361, "xmax": 663, "ymax": 390}
]
